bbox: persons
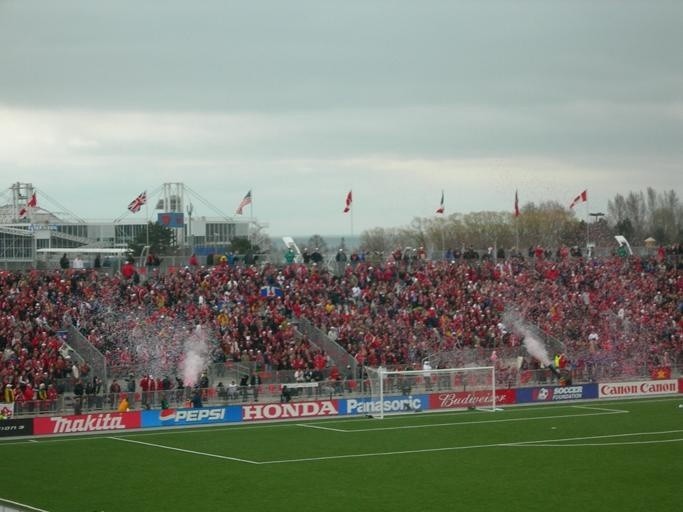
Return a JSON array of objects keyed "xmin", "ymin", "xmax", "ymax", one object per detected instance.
[{"xmin": 1, "ymin": 240, "xmax": 683, "ymax": 415}]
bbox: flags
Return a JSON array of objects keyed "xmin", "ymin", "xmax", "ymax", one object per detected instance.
[
  {"xmin": 127, "ymin": 192, "xmax": 147, "ymax": 213},
  {"xmin": 514, "ymin": 189, "xmax": 520, "ymax": 216},
  {"xmin": 343, "ymin": 192, "xmax": 352, "ymax": 213},
  {"xmin": 436, "ymin": 192, "xmax": 444, "ymax": 213},
  {"xmin": 18, "ymin": 193, "xmax": 37, "ymax": 218},
  {"xmin": 569, "ymin": 190, "xmax": 588, "ymax": 209},
  {"xmin": 235, "ymin": 190, "xmax": 253, "ymax": 215}
]
